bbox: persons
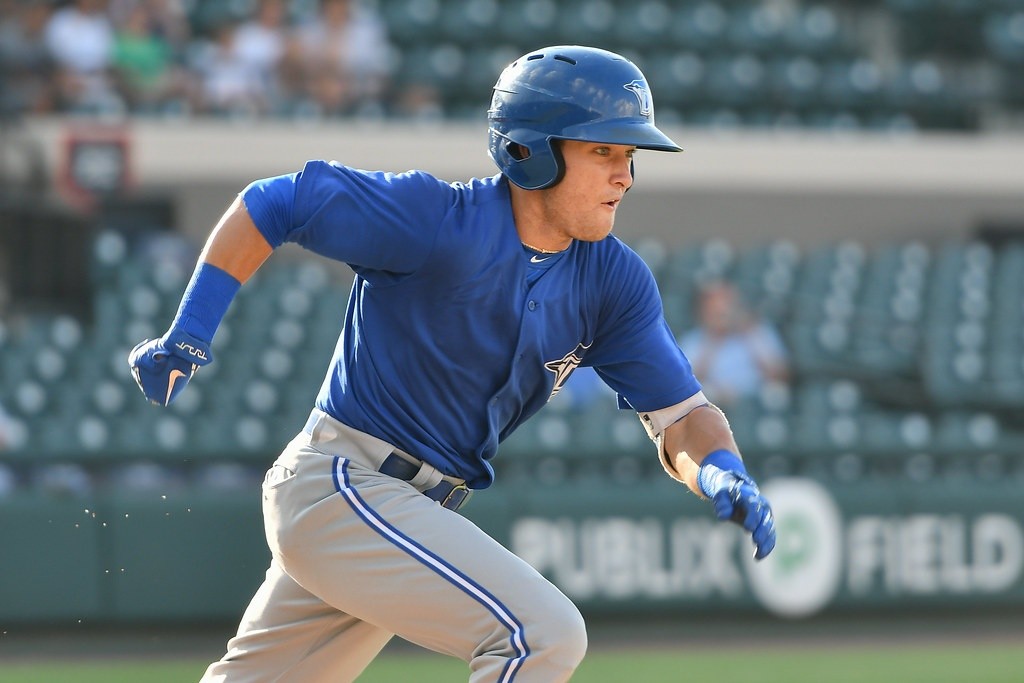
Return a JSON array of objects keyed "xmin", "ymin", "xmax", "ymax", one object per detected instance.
[
  {"xmin": 127, "ymin": 46, "xmax": 777, "ymax": 683},
  {"xmin": 674, "ymin": 276, "xmax": 792, "ymax": 397},
  {"xmin": 0, "ymin": 0, "xmax": 448, "ymax": 119}
]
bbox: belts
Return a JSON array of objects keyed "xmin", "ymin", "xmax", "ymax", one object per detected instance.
[{"xmin": 378, "ymin": 452, "xmax": 470, "ymax": 512}]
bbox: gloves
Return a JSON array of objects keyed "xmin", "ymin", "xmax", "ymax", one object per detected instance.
[
  {"xmin": 696, "ymin": 448, "xmax": 777, "ymax": 562},
  {"xmin": 128, "ymin": 262, "xmax": 244, "ymax": 407}
]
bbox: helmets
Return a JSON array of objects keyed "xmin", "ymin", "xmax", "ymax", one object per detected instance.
[{"xmin": 487, "ymin": 44, "xmax": 685, "ymax": 192}]
